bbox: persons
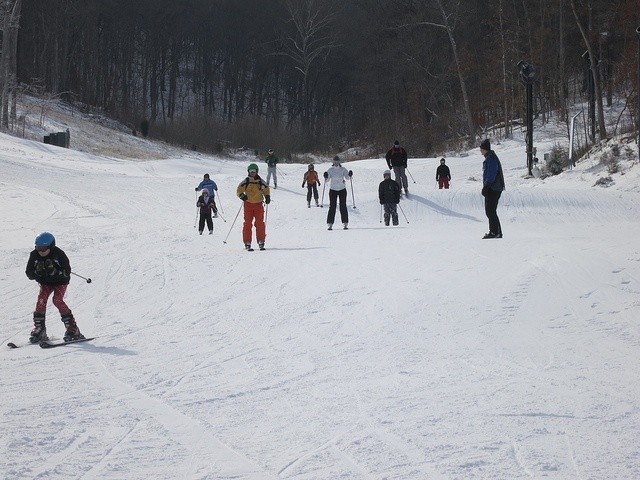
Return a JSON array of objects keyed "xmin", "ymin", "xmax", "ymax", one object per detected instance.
[
  {"xmin": 195, "ymin": 173, "xmax": 218, "ymax": 218},
  {"xmin": 235, "ymin": 164, "xmax": 270, "ymax": 250},
  {"xmin": 435, "ymin": 157, "xmax": 451, "ymax": 189},
  {"xmin": 385, "ymin": 140, "xmax": 408, "ymax": 195},
  {"xmin": 302, "ymin": 163, "xmax": 321, "ymax": 207},
  {"xmin": 480, "ymin": 139, "xmax": 504, "ymax": 238},
  {"xmin": 25, "ymin": 232, "xmax": 86, "ymax": 342},
  {"xmin": 379, "ymin": 170, "xmax": 399, "ymax": 226},
  {"xmin": 324, "ymin": 155, "xmax": 352, "ymax": 230},
  {"xmin": 265, "ymin": 149, "xmax": 279, "ymax": 190},
  {"xmin": 196, "ymin": 188, "xmax": 217, "ymax": 234}
]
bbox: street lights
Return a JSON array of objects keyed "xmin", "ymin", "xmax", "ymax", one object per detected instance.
[{"xmin": 599, "ymin": 33, "xmax": 608, "ymax": 92}]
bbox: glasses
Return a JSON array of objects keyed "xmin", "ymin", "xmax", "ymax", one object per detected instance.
[
  {"xmin": 249, "ymin": 169, "xmax": 256, "ymax": 173},
  {"xmin": 35, "ymin": 243, "xmax": 54, "ymax": 252}
]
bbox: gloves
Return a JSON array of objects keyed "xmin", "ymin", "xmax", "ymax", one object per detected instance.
[
  {"xmin": 240, "ymin": 193, "xmax": 248, "ymax": 201},
  {"xmin": 324, "ymin": 172, "xmax": 328, "ymax": 178},
  {"xmin": 389, "ymin": 165, "xmax": 392, "ymax": 169},
  {"xmin": 33, "ymin": 260, "xmax": 45, "ymax": 278},
  {"xmin": 380, "ymin": 200, "xmax": 384, "ymax": 204},
  {"xmin": 349, "ymin": 170, "xmax": 352, "ymax": 176},
  {"xmin": 246, "ymin": 199, "xmax": 247, "ymax": 200},
  {"xmin": 482, "ymin": 186, "xmax": 489, "ymax": 196},
  {"xmin": 405, "ymin": 163, "xmax": 407, "ymax": 168},
  {"xmin": 44, "ymin": 259, "xmax": 59, "ymax": 278},
  {"xmin": 265, "ymin": 195, "xmax": 270, "ymax": 204},
  {"xmin": 195, "ymin": 188, "xmax": 198, "ymax": 191},
  {"xmin": 395, "ymin": 198, "xmax": 399, "ymax": 203},
  {"xmin": 199, "ymin": 203, "xmax": 205, "ymax": 207},
  {"xmin": 209, "ymin": 203, "xmax": 214, "ymax": 207}
]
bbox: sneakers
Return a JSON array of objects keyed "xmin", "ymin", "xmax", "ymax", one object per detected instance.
[
  {"xmin": 246, "ymin": 243, "xmax": 250, "ymax": 245},
  {"xmin": 343, "ymin": 223, "xmax": 348, "ymax": 229},
  {"xmin": 405, "ymin": 189, "xmax": 408, "ymax": 195},
  {"xmin": 328, "ymin": 224, "xmax": 332, "ymax": 230},
  {"xmin": 315, "ymin": 201, "xmax": 319, "ymax": 206},
  {"xmin": 259, "ymin": 241, "xmax": 264, "ymax": 244},
  {"xmin": 482, "ymin": 231, "xmax": 502, "ymax": 239},
  {"xmin": 307, "ymin": 201, "xmax": 310, "ymax": 205},
  {"xmin": 209, "ymin": 230, "xmax": 213, "ymax": 234},
  {"xmin": 199, "ymin": 231, "xmax": 202, "ymax": 234}
]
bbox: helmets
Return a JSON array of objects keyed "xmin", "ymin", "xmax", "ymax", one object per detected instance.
[
  {"xmin": 35, "ymin": 232, "xmax": 55, "ymax": 252},
  {"xmin": 248, "ymin": 164, "xmax": 258, "ymax": 173}
]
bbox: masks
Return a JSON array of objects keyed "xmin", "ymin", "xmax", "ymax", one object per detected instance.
[
  {"xmin": 203, "ymin": 194, "xmax": 208, "ymax": 196},
  {"xmin": 395, "ymin": 145, "xmax": 399, "ymax": 148},
  {"xmin": 204, "ymin": 178, "xmax": 208, "ymax": 180},
  {"xmin": 385, "ymin": 176, "xmax": 390, "ymax": 179}
]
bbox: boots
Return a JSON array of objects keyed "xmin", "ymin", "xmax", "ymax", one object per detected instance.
[
  {"xmin": 61, "ymin": 315, "xmax": 78, "ymax": 336},
  {"xmin": 31, "ymin": 317, "xmax": 45, "ymax": 336}
]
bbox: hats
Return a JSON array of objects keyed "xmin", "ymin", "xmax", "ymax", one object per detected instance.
[
  {"xmin": 395, "ymin": 141, "xmax": 399, "ymax": 145},
  {"xmin": 333, "ymin": 156, "xmax": 340, "ymax": 160},
  {"xmin": 480, "ymin": 139, "xmax": 490, "ymax": 150},
  {"xmin": 202, "ymin": 188, "xmax": 209, "ymax": 194},
  {"xmin": 383, "ymin": 170, "xmax": 390, "ymax": 178}
]
